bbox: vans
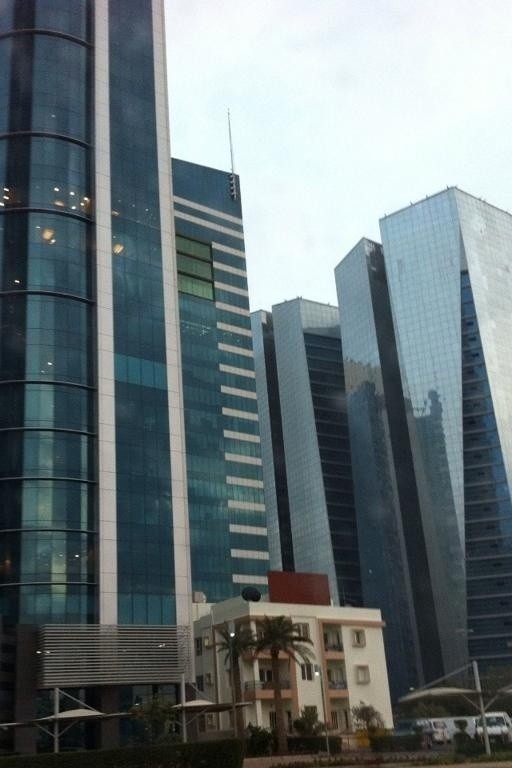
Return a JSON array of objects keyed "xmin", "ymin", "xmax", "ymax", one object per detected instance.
[{"xmin": 395, "ymin": 710, "xmax": 512, "ymax": 743}]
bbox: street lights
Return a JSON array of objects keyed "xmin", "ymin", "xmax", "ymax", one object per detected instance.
[
  {"xmin": 228, "ymin": 631, "xmax": 238, "ymax": 736},
  {"xmin": 314, "ymin": 667, "xmax": 331, "ymax": 756}
]
